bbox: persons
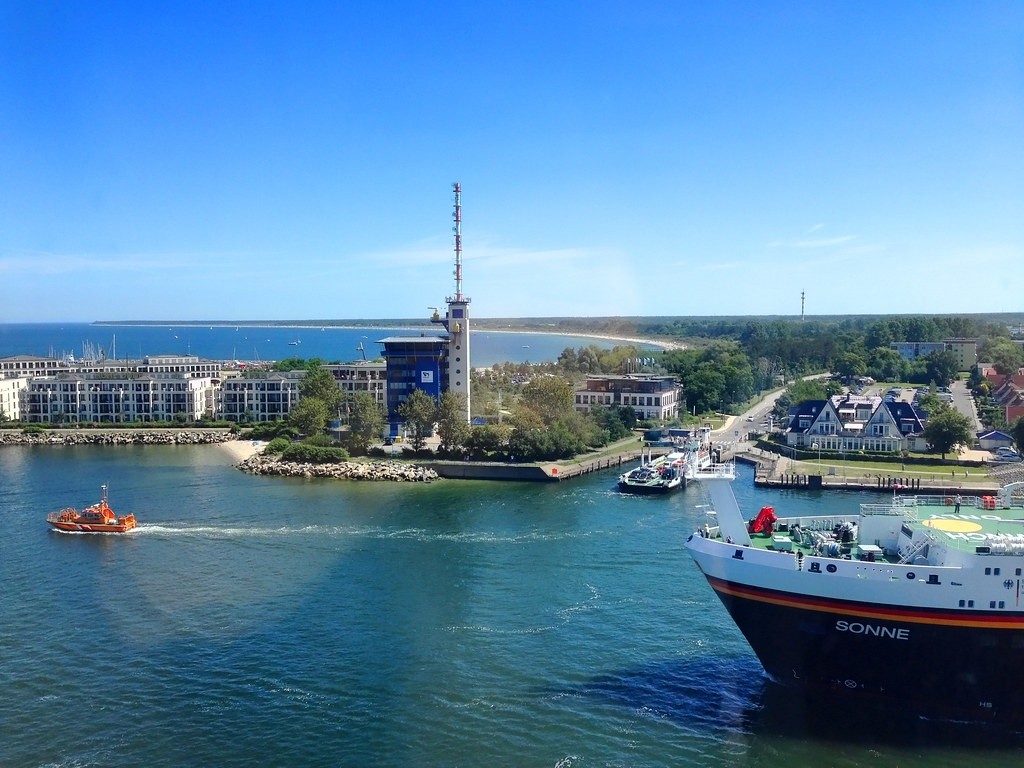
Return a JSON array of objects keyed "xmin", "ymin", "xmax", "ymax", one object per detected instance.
[
  {"xmin": 697, "ymin": 524, "xmax": 709, "ymax": 538},
  {"xmin": 796, "ymin": 548, "xmax": 803, "ymax": 571},
  {"xmin": 953, "ymin": 494, "xmax": 962, "ymax": 513},
  {"xmin": 812, "ymin": 547, "xmax": 823, "ymax": 556},
  {"xmin": 780, "ymin": 547, "xmax": 795, "ymax": 554},
  {"xmin": 726, "ymin": 536, "xmax": 732, "ymax": 543},
  {"xmin": 1000, "ymin": 494, "xmax": 1006, "ymax": 507}
]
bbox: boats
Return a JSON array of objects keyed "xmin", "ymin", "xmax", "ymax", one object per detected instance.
[
  {"xmin": 47, "ymin": 481, "xmax": 138, "ymax": 533},
  {"xmin": 615, "ymin": 450, "xmax": 697, "ymax": 493},
  {"xmin": 683, "ymin": 470, "xmax": 1024, "ymax": 728}
]
